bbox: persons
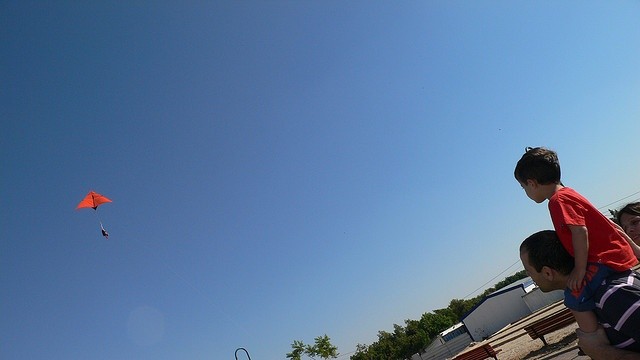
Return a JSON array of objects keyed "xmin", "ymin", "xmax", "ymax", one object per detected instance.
[
  {"xmin": 520, "ymin": 230, "xmax": 638, "ymax": 358},
  {"xmin": 519, "ymin": 146, "xmax": 638, "ymax": 360},
  {"xmin": 608, "ymin": 199, "xmax": 640, "ymax": 257}
]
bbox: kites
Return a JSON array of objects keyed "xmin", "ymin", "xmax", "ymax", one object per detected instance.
[{"xmin": 76, "ymin": 190, "xmax": 113, "ymax": 240}]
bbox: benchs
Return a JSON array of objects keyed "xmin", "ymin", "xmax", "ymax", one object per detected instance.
[
  {"xmin": 524, "ymin": 306, "xmax": 577, "ymax": 345},
  {"xmin": 452, "ymin": 344, "xmax": 503, "ymax": 360}
]
